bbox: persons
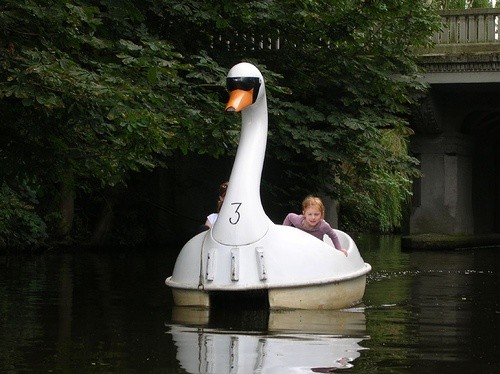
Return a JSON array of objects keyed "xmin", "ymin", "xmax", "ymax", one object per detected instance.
[
  {"xmin": 283, "ymin": 196, "xmax": 348, "ymax": 257},
  {"xmin": 205, "ymin": 183, "xmax": 229, "ymax": 229}
]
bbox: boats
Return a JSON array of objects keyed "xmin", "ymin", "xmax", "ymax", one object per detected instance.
[{"xmin": 163, "ymin": 61, "xmax": 373, "ymax": 312}]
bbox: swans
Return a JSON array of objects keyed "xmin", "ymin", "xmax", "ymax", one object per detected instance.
[{"xmin": 166, "ymin": 62, "xmax": 371, "ymax": 312}]
